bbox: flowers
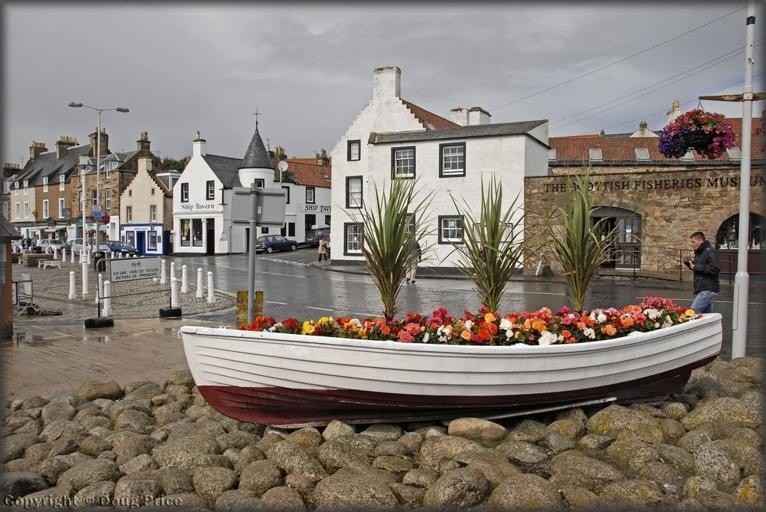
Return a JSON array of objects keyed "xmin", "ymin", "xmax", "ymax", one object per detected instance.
[
  {"xmin": 230, "ymin": 297, "xmax": 696, "ymax": 350},
  {"xmin": 655, "ymin": 107, "xmax": 736, "ymax": 158}
]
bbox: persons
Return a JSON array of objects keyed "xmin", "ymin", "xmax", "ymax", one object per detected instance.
[
  {"xmin": 14, "ymin": 234, "xmax": 38, "ymax": 253},
  {"xmin": 403, "ymin": 236, "xmax": 422, "ymax": 284},
  {"xmin": 318, "ymin": 235, "xmax": 327, "ymax": 264},
  {"xmin": 683, "ymin": 231, "xmax": 721, "ymax": 313}
]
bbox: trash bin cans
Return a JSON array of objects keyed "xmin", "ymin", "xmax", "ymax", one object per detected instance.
[
  {"xmin": 32, "ymin": 246, "xmax": 41, "ymax": 253},
  {"xmin": 94, "ymin": 252, "xmax": 106, "ymax": 272}
]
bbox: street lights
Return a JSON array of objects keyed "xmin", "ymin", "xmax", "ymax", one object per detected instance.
[{"xmin": 68, "ymin": 103, "xmax": 129, "ymax": 258}]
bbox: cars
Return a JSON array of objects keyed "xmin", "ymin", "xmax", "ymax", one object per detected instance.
[
  {"xmin": 305, "ymin": 227, "xmax": 330, "ymax": 248},
  {"xmin": 11, "ymin": 238, "xmax": 140, "ymax": 258},
  {"xmin": 256, "ymin": 235, "xmax": 298, "ymax": 254}
]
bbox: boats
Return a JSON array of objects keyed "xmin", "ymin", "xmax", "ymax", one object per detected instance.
[{"xmin": 178, "ymin": 312, "xmax": 722, "ymax": 430}]
